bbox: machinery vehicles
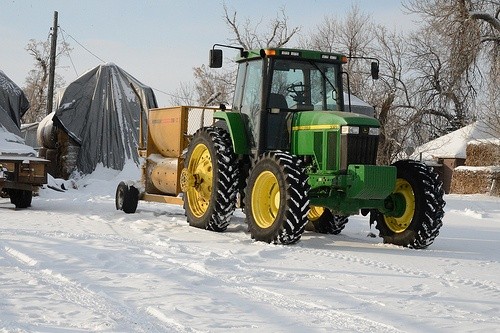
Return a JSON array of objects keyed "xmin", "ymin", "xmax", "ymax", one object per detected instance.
[
  {"xmin": 0, "ymin": 109, "xmax": 83, "ymax": 209},
  {"xmin": 114, "ymin": 42, "xmax": 447, "ymax": 252}
]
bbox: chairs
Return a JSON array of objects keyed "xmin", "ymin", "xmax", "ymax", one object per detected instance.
[{"xmin": 259, "ymin": 92, "xmax": 292, "ymax": 150}]
